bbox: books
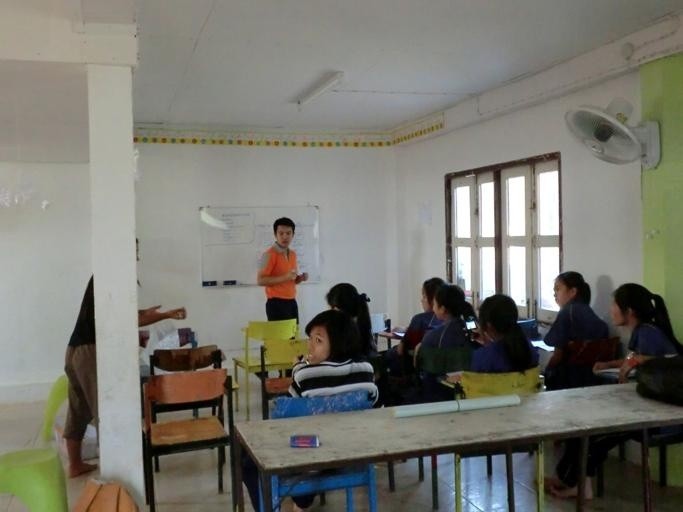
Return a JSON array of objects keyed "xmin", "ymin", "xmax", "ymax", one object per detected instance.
[{"xmin": 593, "ymin": 367, "xmax": 638, "ymax": 384}]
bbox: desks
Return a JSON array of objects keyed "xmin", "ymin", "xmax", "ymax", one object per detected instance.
[{"xmin": 233, "ymin": 379, "xmax": 682, "ymax": 511}]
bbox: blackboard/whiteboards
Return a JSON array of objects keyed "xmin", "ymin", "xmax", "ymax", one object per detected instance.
[{"xmin": 199, "ymin": 206, "xmax": 321, "ymax": 289}]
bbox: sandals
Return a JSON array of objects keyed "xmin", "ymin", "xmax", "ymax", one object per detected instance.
[{"xmin": 532, "ymin": 474, "xmax": 579, "ymax": 501}]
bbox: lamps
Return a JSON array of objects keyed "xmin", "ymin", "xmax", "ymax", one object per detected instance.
[{"xmin": 294, "ymin": 68, "xmax": 344, "ymax": 107}]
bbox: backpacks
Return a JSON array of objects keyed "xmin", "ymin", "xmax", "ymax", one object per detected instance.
[
  {"xmin": 71, "ymin": 478, "xmax": 138, "ymax": 511},
  {"xmin": 379, "ymin": 365, "xmax": 456, "ymax": 406},
  {"xmin": 635, "ymin": 354, "xmax": 683, "ymax": 406}
]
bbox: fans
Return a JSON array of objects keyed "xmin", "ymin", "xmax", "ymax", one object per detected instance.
[{"xmin": 562, "ymin": 95, "xmax": 661, "ymax": 172}]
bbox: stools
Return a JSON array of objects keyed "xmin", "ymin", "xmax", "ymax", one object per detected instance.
[
  {"xmin": 1, "ymin": 448, "xmax": 72, "ymax": 511},
  {"xmin": 41, "ymin": 372, "xmax": 70, "ymax": 448}
]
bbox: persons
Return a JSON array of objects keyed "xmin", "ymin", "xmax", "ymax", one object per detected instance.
[
  {"xmin": 254, "ymin": 217, "xmax": 309, "ymax": 379},
  {"xmin": 381, "ymin": 276, "xmax": 448, "ymax": 362},
  {"xmin": 533, "ymin": 282, "xmax": 682, "ymax": 500},
  {"xmin": 443, "ymin": 293, "xmax": 540, "ymax": 400},
  {"xmin": 61, "ymin": 236, "xmax": 188, "ymax": 479},
  {"xmin": 323, "ymin": 282, "xmax": 377, "ymax": 356},
  {"xmin": 403, "ymin": 282, "xmax": 479, "ymax": 375},
  {"xmin": 285, "ymin": 308, "xmax": 381, "ymax": 410},
  {"xmin": 540, "ymin": 270, "xmax": 608, "ymax": 388}
]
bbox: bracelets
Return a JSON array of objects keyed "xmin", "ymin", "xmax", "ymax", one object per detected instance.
[{"xmin": 624, "ymin": 356, "xmax": 640, "ymax": 369}]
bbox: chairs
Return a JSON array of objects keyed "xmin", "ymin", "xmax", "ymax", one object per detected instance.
[
  {"xmin": 139, "ymin": 328, "xmax": 198, "ymax": 416},
  {"xmin": 145, "ymin": 344, "xmax": 224, "ymax": 450},
  {"xmin": 232, "ymin": 319, "xmax": 298, "ymax": 420},
  {"xmin": 431, "ymin": 365, "xmax": 545, "ymax": 512},
  {"xmin": 142, "ymin": 368, "xmax": 233, "ymax": 512},
  {"xmin": 257, "ymin": 388, "xmax": 378, "ymax": 511},
  {"xmin": 259, "ymin": 338, "xmax": 308, "ymax": 420}
]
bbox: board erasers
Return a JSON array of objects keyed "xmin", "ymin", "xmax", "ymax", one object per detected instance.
[
  {"xmin": 202, "ymin": 280, "xmax": 217, "ymax": 286},
  {"xmin": 223, "ymin": 280, "xmax": 236, "ymax": 285}
]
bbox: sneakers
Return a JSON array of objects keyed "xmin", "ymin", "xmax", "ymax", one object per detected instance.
[{"xmin": 585, "ymin": 477, "xmax": 594, "ymax": 498}]
